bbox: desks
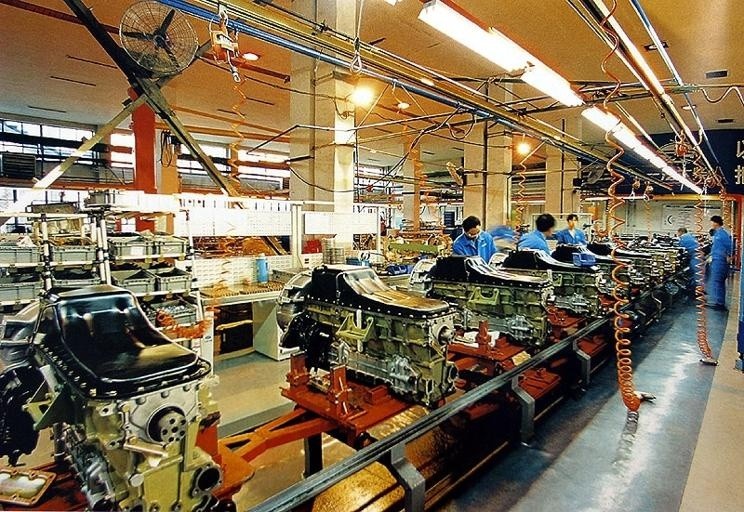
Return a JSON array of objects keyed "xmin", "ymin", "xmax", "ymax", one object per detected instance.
[{"xmin": 197, "ymin": 272, "xmax": 311, "ymax": 368}]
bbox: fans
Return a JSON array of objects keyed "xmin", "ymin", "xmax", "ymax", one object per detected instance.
[
  {"xmin": 114, "ymin": 0, "xmax": 202, "ymax": 81},
  {"xmin": 446, "ymin": 158, "xmax": 474, "ymax": 191}
]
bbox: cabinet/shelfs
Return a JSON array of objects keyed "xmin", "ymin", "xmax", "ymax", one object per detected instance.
[{"xmin": 3, "ymin": 212, "xmax": 196, "ymax": 345}]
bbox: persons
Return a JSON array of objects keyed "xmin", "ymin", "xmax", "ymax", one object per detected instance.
[
  {"xmin": 519, "ymin": 214, "xmax": 555, "ymax": 256},
  {"xmin": 559, "ymin": 214, "xmax": 587, "ymax": 248},
  {"xmin": 704, "ymin": 215, "xmax": 736, "ymax": 309},
  {"xmin": 676, "ymin": 228, "xmax": 706, "ymax": 297},
  {"xmin": 451, "ymin": 216, "xmax": 499, "ymax": 264},
  {"xmin": 380, "ymin": 217, "xmax": 387, "ymax": 235}
]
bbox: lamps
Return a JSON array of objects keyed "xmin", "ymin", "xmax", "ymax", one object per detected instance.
[
  {"xmin": 581, "ymin": 98, "xmax": 704, "ymax": 196},
  {"xmin": 416, "ymin": 1, "xmax": 585, "ymax": 110}
]
